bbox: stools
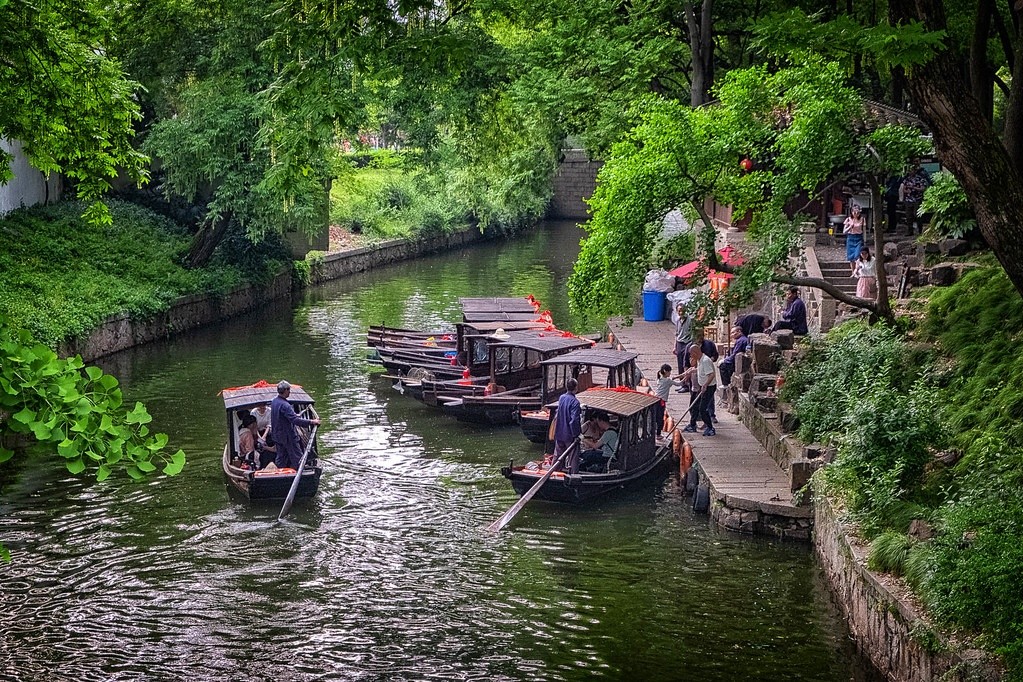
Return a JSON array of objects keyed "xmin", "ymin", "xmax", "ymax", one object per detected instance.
[{"xmin": 704, "ymin": 326, "xmax": 717, "ymax": 342}]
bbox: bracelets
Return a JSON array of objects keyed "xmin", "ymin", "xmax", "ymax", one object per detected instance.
[{"xmin": 864, "ymin": 242, "xmax": 867, "ymax": 243}]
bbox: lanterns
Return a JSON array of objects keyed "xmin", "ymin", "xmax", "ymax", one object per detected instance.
[{"xmin": 740, "ymin": 159, "xmax": 752, "ymax": 169}]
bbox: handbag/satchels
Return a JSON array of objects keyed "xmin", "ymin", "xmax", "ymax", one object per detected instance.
[{"xmin": 549, "ymin": 412, "xmax": 558, "ymax": 440}]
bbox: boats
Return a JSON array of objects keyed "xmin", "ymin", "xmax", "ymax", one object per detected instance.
[
  {"xmin": 512, "ymin": 348, "xmax": 643, "ymax": 445},
  {"xmin": 499, "ymin": 387, "xmax": 674, "ymax": 503},
  {"xmin": 365, "ymin": 294, "xmax": 596, "ymax": 425},
  {"xmin": 222, "ymin": 380, "xmax": 324, "ymax": 500}
]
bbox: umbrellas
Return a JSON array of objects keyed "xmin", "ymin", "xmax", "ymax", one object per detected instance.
[{"xmin": 670, "ymin": 244, "xmax": 748, "ymax": 354}]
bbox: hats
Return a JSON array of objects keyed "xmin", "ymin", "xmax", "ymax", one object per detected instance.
[
  {"xmin": 277, "ymin": 380, "xmax": 291, "ymax": 388},
  {"xmin": 859, "ymin": 246, "xmax": 869, "ymax": 253}
]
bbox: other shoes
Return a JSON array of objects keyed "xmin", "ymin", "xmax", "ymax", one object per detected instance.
[
  {"xmin": 905, "ymin": 233, "xmax": 914, "ymax": 237},
  {"xmin": 674, "ymin": 385, "xmax": 682, "ymax": 391},
  {"xmin": 697, "ymin": 414, "xmax": 702, "ymax": 420},
  {"xmin": 711, "ymin": 417, "xmax": 718, "ymax": 423},
  {"xmin": 703, "ymin": 427, "xmax": 716, "ymax": 436},
  {"xmin": 656, "ymin": 436, "xmax": 667, "ymax": 442},
  {"xmin": 698, "ymin": 423, "xmax": 708, "ymax": 429},
  {"xmin": 678, "ymin": 387, "xmax": 689, "ymax": 393},
  {"xmin": 683, "ymin": 424, "xmax": 698, "ymax": 433},
  {"xmin": 719, "ymin": 384, "xmax": 730, "ymax": 388}
]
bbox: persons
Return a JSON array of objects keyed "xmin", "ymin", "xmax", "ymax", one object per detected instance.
[
  {"xmin": 773, "ymin": 286, "xmax": 808, "ymax": 336},
  {"xmin": 682, "ymin": 345, "xmax": 717, "ymax": 436},
  {"xmin": 236, "ymin": 410, "xmax": 276, "ymax": 469},
  {"xmin": 741, "ymin": 314, "xmax": 772, "ymax": 337},
  {"xmin": 883, "ymin": 157, "xmax": 931, "ymax": 235},
  {"xmin": 653, "ymin": 364, "xmax": 682, "ymax": 439},
  {"xmin": 843, "ymin": 205, "xmax": 867, "ymax": 278},
  {"xmin": 831, "ymin": 182, "xmax": 844, "ymax": 213},
  {"xmin": 850, "ymin": 246, "xmax": 875, "ymax": 297},
  {"xmin": 580, "ymin": 412, "xmax": 618, "ymax": 466},
  {"xmin": 249, "ymin": 405, "xmax": 271, "ymax": 442},
  {"xmin": 673, "ymin": 303, "xmax": 691, "ymax": 392},
  {"xmin": 683, "ymin": 327, "xmax": 719, "ymax": 370},
  {"xmin": 717, "ymin": 326, "xmax": 747, "ymax": 385},
  {"xmin": 555, "ymin": 378, "xmax": 580, "ymax": 474},
  {"xmin": 270, "ymin": 380, "xmax": 320, "ymax": 469}
]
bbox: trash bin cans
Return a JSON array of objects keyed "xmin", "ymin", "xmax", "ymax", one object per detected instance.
[{"xmin": 642, "ymin": 287, "xmax": 667, "ymax": 322}]
